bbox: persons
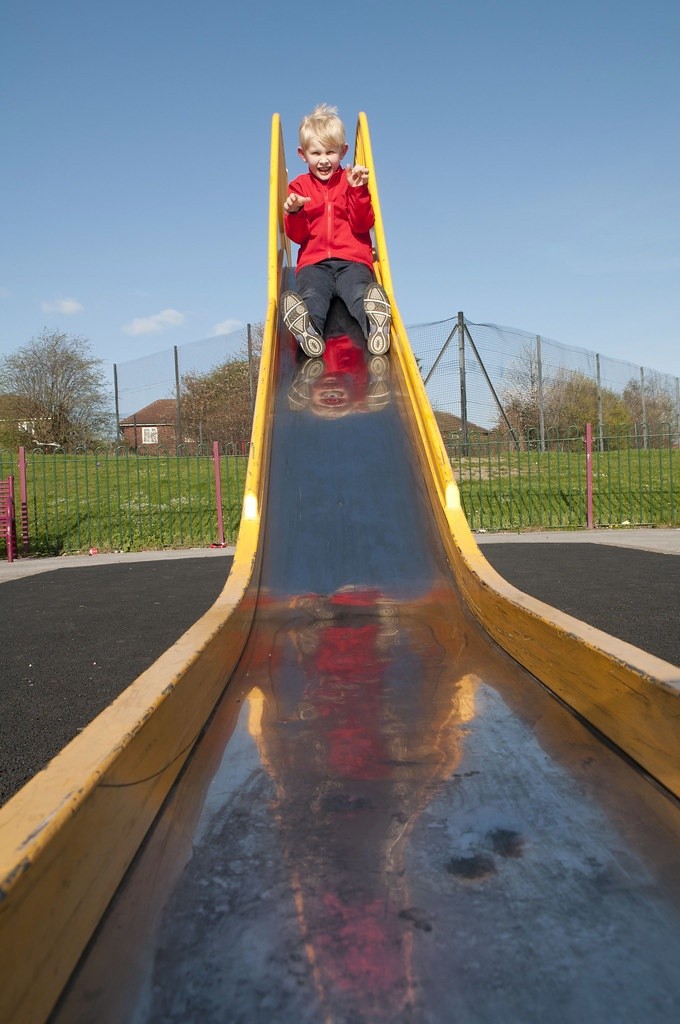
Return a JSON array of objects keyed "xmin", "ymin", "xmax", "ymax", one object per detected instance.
[
  {"xmin": 286, "ymin": 294, "xmax": 389, "ymax": 417},
  {"xmin": 281, "ymin": 103, "xmax": 391, "ymax": 357}
]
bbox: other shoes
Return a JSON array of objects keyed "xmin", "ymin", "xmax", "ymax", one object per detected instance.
[
  {"xmin": 362, "ymin": 282, "xmax": 391, "ymax": 355},
  {"xmin": 366, "ymin": 355, "xmax": 390, "ymax": 412},
  {"xmin": 287, "ymin": 358, "xmax": 324, "ymax": 413},
  {"xmin": 280, "ymin": 290, "xmax": 326, "ymax": 358}
]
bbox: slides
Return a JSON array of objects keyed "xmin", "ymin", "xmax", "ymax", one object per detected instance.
[{"xmin": 0, "ymin": 112, "xmax": 677, "ymax": 1024}]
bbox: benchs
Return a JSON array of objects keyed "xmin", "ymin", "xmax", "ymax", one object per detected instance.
[{"xmin": 0, "ymin": 474, "xmax": 18, "ymax": 563}]
